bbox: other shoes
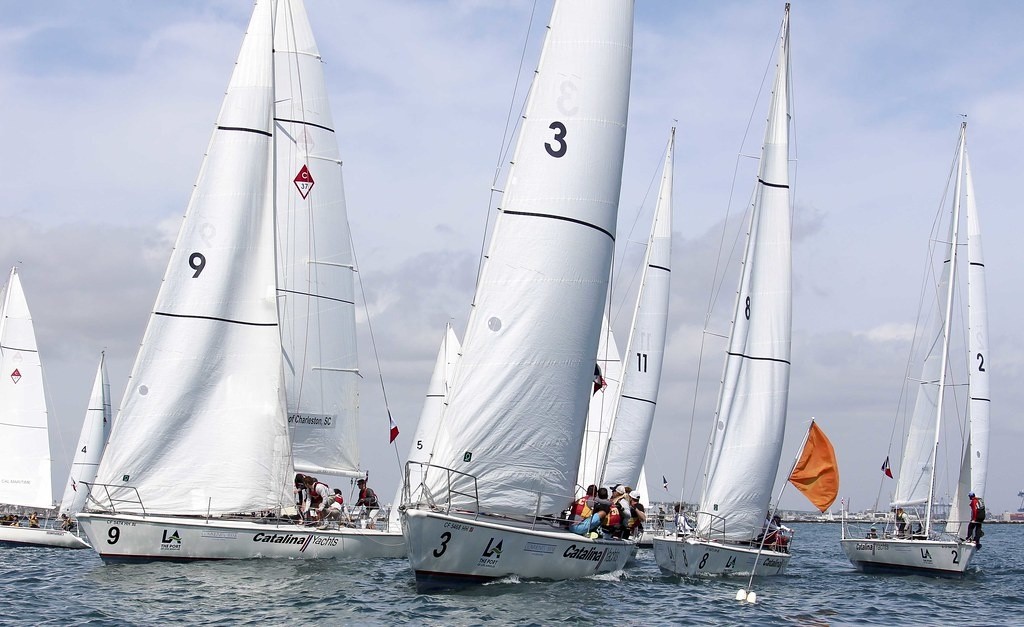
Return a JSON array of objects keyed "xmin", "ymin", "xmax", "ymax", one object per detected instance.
[{"xmin": 585, "ymin": 532, "xmax": 598, "ymax": 539}]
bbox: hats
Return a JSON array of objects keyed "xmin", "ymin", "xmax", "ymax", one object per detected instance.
[
  {"xmin": 356, "ymin": 479, "xmax": 366, "ymax": 485},
  {"xmin": 629, "ymin": 491, "xmax": 641, "ymax": 499},
  {"xmin": 610, "ymin": 484, "xmax": 625, "ymax": 494},
  {"xmin": 968, "ymin": 492, "xmax": 975, "ymax": 496}
]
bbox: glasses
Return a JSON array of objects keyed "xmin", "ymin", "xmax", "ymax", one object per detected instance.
[{"xmin": 612, "ymin": 490, "xmax": 615, "ymax": 493}]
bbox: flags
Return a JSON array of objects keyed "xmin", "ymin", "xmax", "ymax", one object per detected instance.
[{"xmin": 789, "ymin": 422, "xmax": 839, "ymax": 513}]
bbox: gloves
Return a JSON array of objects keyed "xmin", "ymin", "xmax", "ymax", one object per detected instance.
[{"xmin": 615, "ymin": 503, "xmax": 624, "ymax": 511}]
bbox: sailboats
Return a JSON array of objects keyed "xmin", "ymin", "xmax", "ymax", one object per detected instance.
[
  {"xmin": 61, "ymin": 0, "xmax": 682, "ymax": 591},
  {"xmin": 0, "ymin": 260, "xmax": 94, "ymax": 550},
  {"xmin": 838, "ymin": 111, "xmax": 992, "ymax": 582},
  {"xmin": 650, "ymin": 1, "xmax": 793, "ymax": 581}
]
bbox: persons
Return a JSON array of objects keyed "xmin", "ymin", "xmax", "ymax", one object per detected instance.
[
  {"xmin": 658, "ymin": 508, "xmax": 665, "ymax": 527},
  {"xmin": 294, "ymin": 474, "xmax": 354, "ymax": 529},
  {"xmin": 894, "ymin": 508, "xmax": 910, "ymax": 532},
  {"xmin": 61, "ymin": 514, "xmax": 73, "ymax": 530},
  {"xmin": 356, "ymin": 479, "xmax": 380, "ymax": 528},
  {"xmin": 569, "ymin": 485, "xmax": 612, "ymax": 534},
  {"xmin": 29, "ymin": 512, "xmax": 38, "ymax": 528},
  {"xmin": 674, "ymin": 505, "xmax": 693, "ymax": 536},
  {"xmin": 965, "ymin": 492, "xmax": 985, "ymax": 549},
  {"xmin": 0, "ymin": 513, "xmax": 20, "ymax": 526},
  {"xmin": 871, "ymin": 527, "xmax": 880, "ymax": 538},
  {"xmin": 597, "ymin": 484, "xmax": 646, "ymax": 538},
  {"xmin": 740, "ymin": 511, "xmax": 794, "ymax": 553}
]
